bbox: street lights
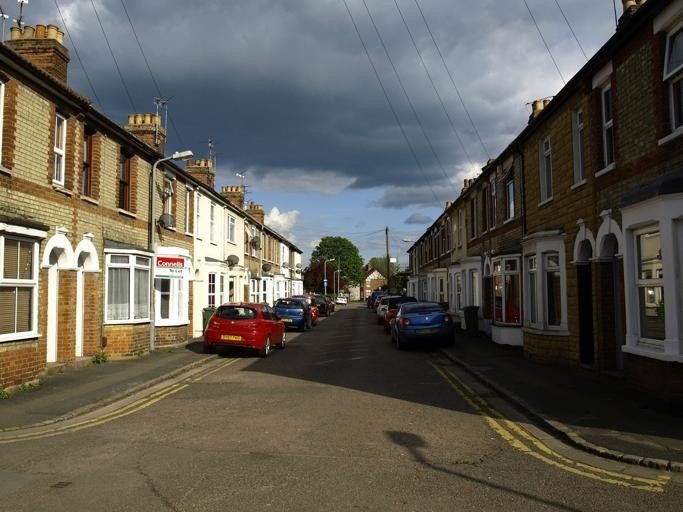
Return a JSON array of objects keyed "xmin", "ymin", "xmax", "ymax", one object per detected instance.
[
  {"xmin": 323, "ymin": 255, "xmax": 354, "ymax": 304},
  {"xmin": 149, "ymin": 149, "xmax": 194, "ymax": 355}
]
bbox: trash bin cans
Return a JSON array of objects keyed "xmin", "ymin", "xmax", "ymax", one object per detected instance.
[
  {"xmin": 463, "ymin": 306, "xmax": 480, "ymax": 330},
  {"xmin": 203, "ymin": 307, "xmax": 216, "ymax": 336}
]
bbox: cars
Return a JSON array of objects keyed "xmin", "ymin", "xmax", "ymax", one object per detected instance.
[
  {"xmin": 272, "ymin": 295, "xmax": 335, "ymax": 331},
  {"xmin": 367, "ymin": 290, "xmax": 456, "ymax": 351},
  {"xmin": 203, "ymin": 301, "xmax": 286, "ymax": 358}
]
